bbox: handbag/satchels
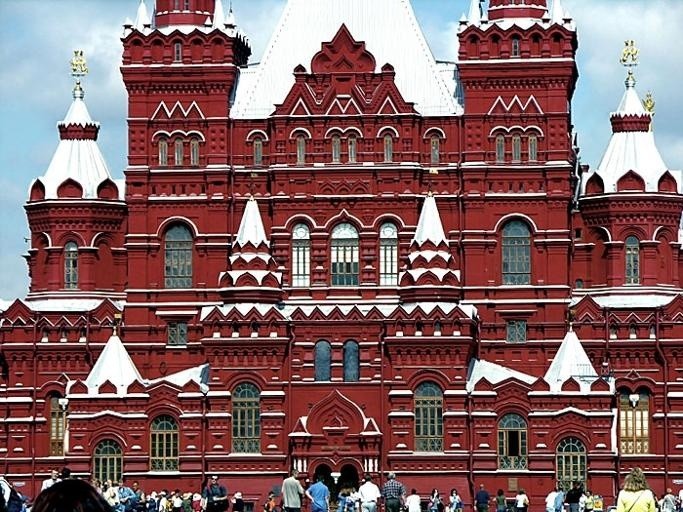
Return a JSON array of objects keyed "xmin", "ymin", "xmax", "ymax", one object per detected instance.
[
  {"xmin": 523, "ymin": 499, "xmax": 529, "ymax": 507},
  {"xmin": 427, "ymin": 502, "xmax": 433, "ymax": 508},
  {"xmin": 214, "ymin": 499, "xmax": 229, "ymax": 512}
]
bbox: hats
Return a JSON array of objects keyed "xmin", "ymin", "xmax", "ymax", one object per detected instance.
[
  {"xmin": 234, "ymin": 492, "xmax": 242, "ymax": 499},
  {"xmin": 157, "ymin": 491, "xmax": 201, "ymax": 502}
]
